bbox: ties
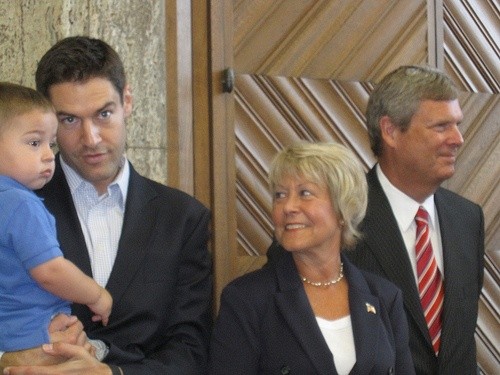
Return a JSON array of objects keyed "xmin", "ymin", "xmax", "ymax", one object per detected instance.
[{"xmin": 415, "ymin": 206, "xmax": 445, "ymax": 357}]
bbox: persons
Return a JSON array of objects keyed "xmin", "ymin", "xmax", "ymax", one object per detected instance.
[
  {"xmin": 210, "ymin": 140, "xmax": 415, "ymax": 375},
  {"xmin": 265, "ymin": 65, "xmax": 485, "ymax": 375},
  {"xmin": 0, "ymin": 81, "xmax": 113, "ymax": 361},
  {"xmin": 0, "ymin": 36, "xmax": 214, "ymax": 375}
]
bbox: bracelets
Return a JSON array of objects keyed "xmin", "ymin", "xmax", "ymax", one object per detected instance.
[{"xmin": 109, "ymin": 363, "xmax": 121, "ymax": 375}]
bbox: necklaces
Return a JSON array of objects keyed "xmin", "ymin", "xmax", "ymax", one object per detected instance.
[{"xmin": 299, "ymin": 261, "xmax": 344, "ymax": 287}]
